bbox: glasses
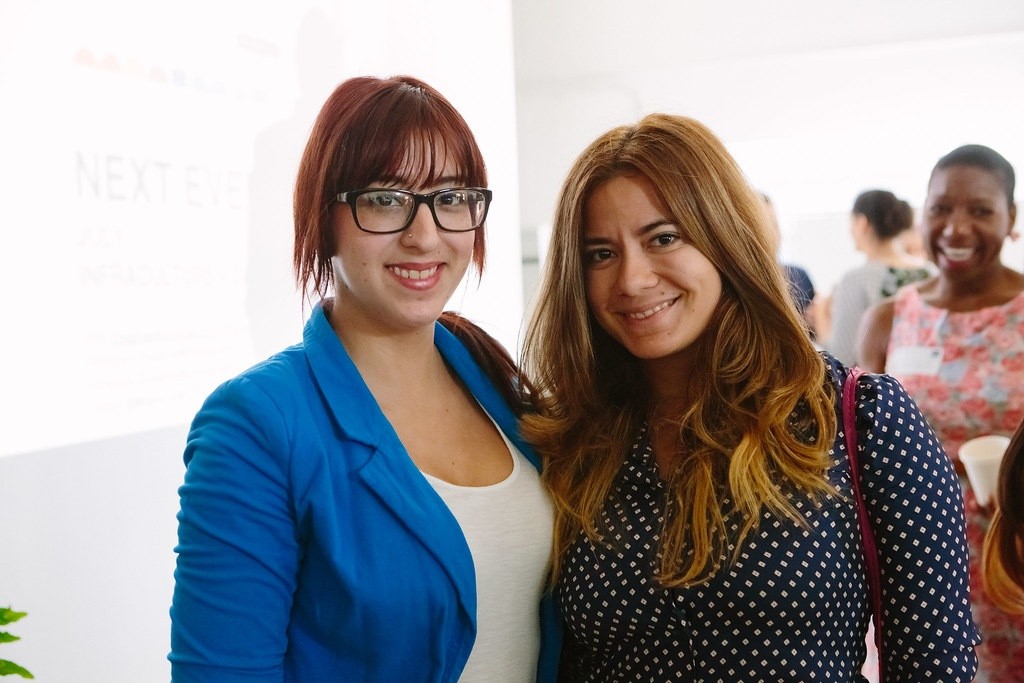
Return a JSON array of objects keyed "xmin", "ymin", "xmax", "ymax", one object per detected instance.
[{"xmin": 321, "ymin": 186, "xmax": 492, "ymax": 235}]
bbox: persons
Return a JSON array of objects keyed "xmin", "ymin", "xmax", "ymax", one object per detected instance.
[
  {"xmin": 166, "ymin": 75, "xmax": 556, "ymax": 683},
  {"xmin": 858, "ymin": 144, "xmax": 1024, "ymax": 683},
  {"xmin": 519, "ymin": 113, "xmax": 984, "ymax": 683},
  {"xmin": 761, "ymin": 189, "xmax": 932, "ymax": 369}
]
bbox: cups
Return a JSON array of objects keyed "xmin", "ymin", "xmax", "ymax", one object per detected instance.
[{"xmin": 958, "ymin": 436, "xmax": 1011, "ymax": 507}]
